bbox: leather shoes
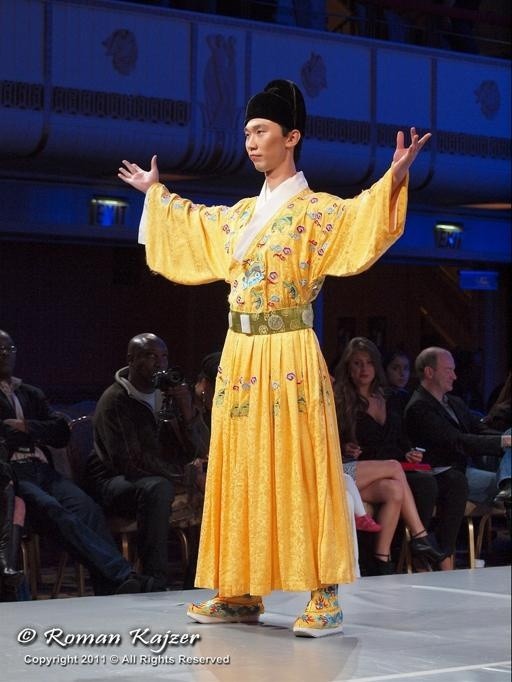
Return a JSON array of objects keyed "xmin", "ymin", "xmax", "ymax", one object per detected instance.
[
  {"xmin": 128, "ymin": 573, "xmax": 154, "ymax": 591},
  {"xmin": 495, "ymin": 484, "xmax": 512, "ymax": 501},
  {"xmin": 95, "ymin": 578, "xmax": 140, "ymax": 595}
]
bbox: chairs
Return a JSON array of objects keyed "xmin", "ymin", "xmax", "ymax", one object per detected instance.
[{"xmin": 20, "ymin": 400, "xmax": 506, "ymax": 600}]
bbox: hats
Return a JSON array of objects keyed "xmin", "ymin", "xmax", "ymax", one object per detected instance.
[{"xmin": 244, "ymin": 78, "xmax": 307, "ymax": 128}]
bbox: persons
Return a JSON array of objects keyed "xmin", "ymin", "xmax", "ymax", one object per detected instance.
[
  {"xmin": 118, "ymin": 80, "xmax": 431, "ymax": 639},
  {"xmin": 0, "ymin": 331, "xmax": 155, "ymax": 596},
  {"xmin": 0, "ymin": 441, "xmax": 27, "ymax": 601},
  {"xmin": 94, "ymin": 331, "xmax": 213, "ymax": 591},
  {"xmin": 324, "ymin": 337, "xmax": 510, "ymax": 577},
  {"xmin": 160, "ymin": 352, "xmax": 221, "ymax": 462}
]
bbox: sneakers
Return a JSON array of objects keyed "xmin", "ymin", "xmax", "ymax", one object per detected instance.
[{"xmin": 355, "ymin": 514, "xmax": 380, "ymax": 532}]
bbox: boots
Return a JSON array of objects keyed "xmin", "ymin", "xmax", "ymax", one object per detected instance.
[
  {"xmin": 293, "ymin": 584, "xmax": 344, "ymax": 638},
  {"xmin": 189, "ymin": 592, "xmax": 263, "ymax": 624}
]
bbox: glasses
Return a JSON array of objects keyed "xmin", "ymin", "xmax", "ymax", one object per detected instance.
[{"xmin": 2, "ymin": 344, "xmax": 18, "ymax": 356}]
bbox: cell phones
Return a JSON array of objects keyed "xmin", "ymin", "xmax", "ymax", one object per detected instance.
[{"xmin": 414, "ymin": 446, "xmax": 426, "ymax": 454}]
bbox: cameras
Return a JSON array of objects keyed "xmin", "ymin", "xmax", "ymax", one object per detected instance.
[{"xmin": 150, "ymin": 368, "xmax": 182, "ymax": 392}]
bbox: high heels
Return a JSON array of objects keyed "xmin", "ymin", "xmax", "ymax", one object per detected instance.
[
  {"xmin": 368, "ymin": 552, "xmax": 390, "ymax": 575},
  {"xmin": 412, "ymin": 539, "xmax": 448, "ymax": 567}
]
